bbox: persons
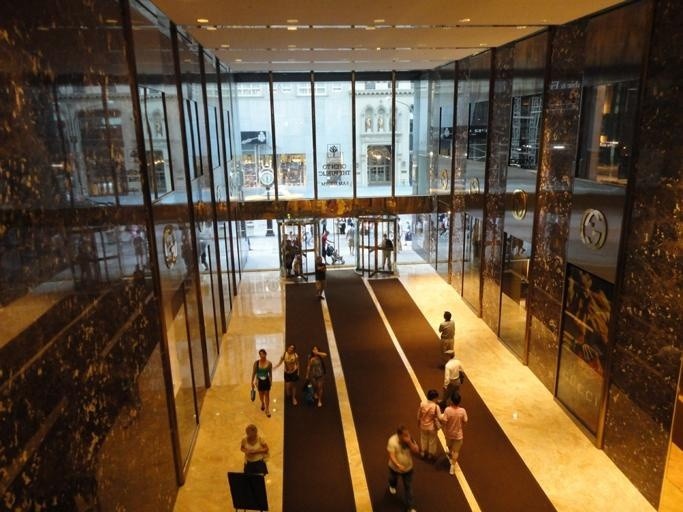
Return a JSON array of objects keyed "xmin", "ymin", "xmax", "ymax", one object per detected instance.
[
  {"xmin": 278, "ymin": 218, "xmax": 413, "ymax": 300},
  {"xmin": 240, "ymin": 424, "xmax": 269, "ymax": 476},
  {"xmin": 273, "ymin": 344, "xmax": 300, "ymax": 405},
  {"xmin": 441, "ymin": 392, "xmax": 468, "ymax": 475},
  {"xmin": 385, "ymin": 426, "xmax": 419, "ymax": 512},
  {"xmin": 304, "ymin": 345, "xmax": 327, "ymax": 407},
  {"xmin": 438, "ymin": 214, "xmax": 448, "ymax": 238},
  {"xmin": 438, "ymin": 349, "xmax": 464, "ymax": 403},
  {"xmin": 439, "ymin": 310, "xmax": 455, "ymax": 368},
  {"xmin": 416, "ymin": 389, "xmax": 441, "ymax": 461},
  {"xmin": 249, "ymin": 349, "xmax": 272, "ymax": 418}
]
